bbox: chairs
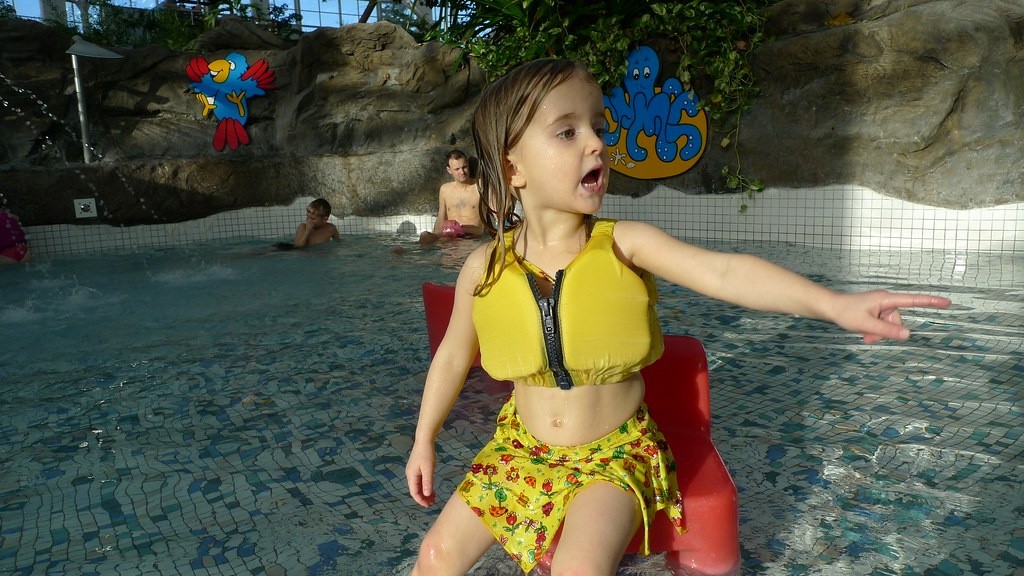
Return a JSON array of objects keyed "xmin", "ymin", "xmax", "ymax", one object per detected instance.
[
  {"xmin": 421, "ymin": 281, "xmax": 514, "ymax": 395},
  {"xmin": 533, "ymin": 334, "xmax": 744, "ymax": 576}
]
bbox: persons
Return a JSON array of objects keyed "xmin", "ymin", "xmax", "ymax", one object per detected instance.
[
  {"xmin": 405, "ymin": 58, "xmax": 953, "ymax": 576},
  {"xmin": 421, "ymin": 150, "xmax": 499, "ymax": 243},
  {"xmin": 294, "ymin": 198, "xmax": 339, "ymax": 246},
  {"xmin": 0, "ymin": 195, "xmax": 31, "ymax": 265}
]
